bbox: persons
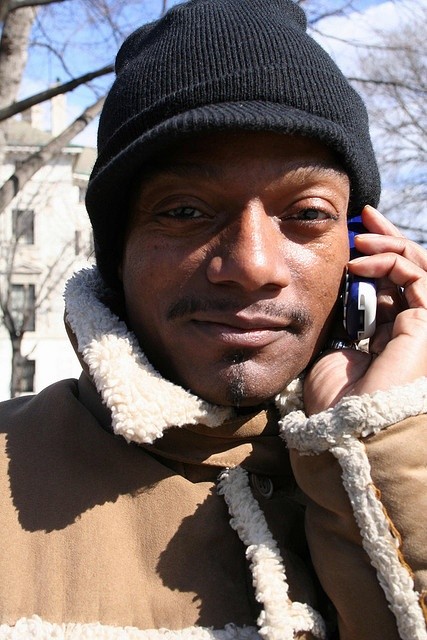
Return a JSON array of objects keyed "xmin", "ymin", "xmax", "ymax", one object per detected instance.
[{"xmin": 0, "ymin": 0, "xmax": 427, "ymax": 637}]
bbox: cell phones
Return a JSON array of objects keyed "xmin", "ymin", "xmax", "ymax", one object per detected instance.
[{"xmin": 334, "ymin": 209, "xmax": 378, "ymax": 345}]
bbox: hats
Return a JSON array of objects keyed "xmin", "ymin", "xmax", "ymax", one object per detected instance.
[{"xmin": 86, "ymin": 0, "xmax": 381, "ymax": 292}]
